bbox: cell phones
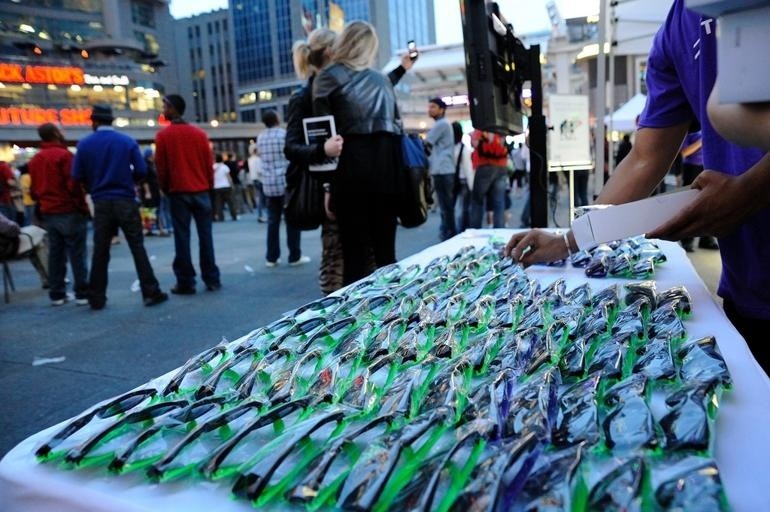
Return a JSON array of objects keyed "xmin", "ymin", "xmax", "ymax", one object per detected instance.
[{"xmin": 408, "ymin": 40, "xmax": 417, "ymax": 60}]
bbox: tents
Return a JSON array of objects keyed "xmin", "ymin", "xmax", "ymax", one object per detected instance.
[{"xmin": 604, "ymin": 93, "xmax": 648, "ymax": 130}]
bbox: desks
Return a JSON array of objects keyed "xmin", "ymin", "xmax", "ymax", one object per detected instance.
[{"xmin": 0, "ymin": 227, "xmax": 770, "ymax": 511}]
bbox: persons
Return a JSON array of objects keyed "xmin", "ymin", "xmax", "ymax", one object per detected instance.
[
  {"xmin": 563, "ymin": 125, "xmax": 610, "ymax": 218},
  {"xmin": 30, "ymin": 125, "xmax": 94, "ymax": 304},
  {"xmin": 284, "ymin": 29, "xmax": 419, "ymax": 297},
  {"xmin": 258, "ymin": 111, "xmax": 310, "ymax": 266},
  {"xmin": 506, "ymin": 0, "xmax": 770, "ymax": 374},
  {"xmin": 312, "ymin": 21, "xmax": 404, "ymax": 286},
  {"xmin": 213, "ymin": 145, "xmax": 268, "ymax": 222},
  {"xmin": 154, "ymin": 94, "xmax": 221, "ymax": 293},
  {"xmin": 112, "ymin": 147, "xmax": 173, "ymax": 243},
  {"xmin": 73, "ymin": 104, "xmax": 169, "ymax": 308},
  {"xmin": 0, "ymin": 161, "xmax": 41, "ymax": 225},
  {"xmin": 616, "ymin": 113, "xmax": 719, "ymax": 252},
  {"xmin": 426, "ymin": 97, "xmax": 529, "ymax": 240}
]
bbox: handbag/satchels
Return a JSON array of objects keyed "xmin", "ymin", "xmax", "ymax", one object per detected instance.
[
  {"xmin": 283, "ymin": 167, "xmax": 322, "ymax": 229},
  {"xmin": 400, "ymin": 134, "xmax": 432, "ymax": 228}
]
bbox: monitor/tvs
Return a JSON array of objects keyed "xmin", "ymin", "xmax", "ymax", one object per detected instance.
[{"xmin": 459, "ymin": 0, "xmax": 525, "ymax": 135}]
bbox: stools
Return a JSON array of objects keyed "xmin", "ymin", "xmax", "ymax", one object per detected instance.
[{"xmin": 0, "ymin": 231, "xmax": 50, "ymax": 305}]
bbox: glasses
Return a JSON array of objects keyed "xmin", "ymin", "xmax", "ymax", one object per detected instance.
[{"xmin": 36, "ymin": 234, "xmax": 732, "ymax": 512}]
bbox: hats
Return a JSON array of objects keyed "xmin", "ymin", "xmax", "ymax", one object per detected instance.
[{"xmin": 88, "ymin": 104, "xmax": 115, "ymax": 122}]
